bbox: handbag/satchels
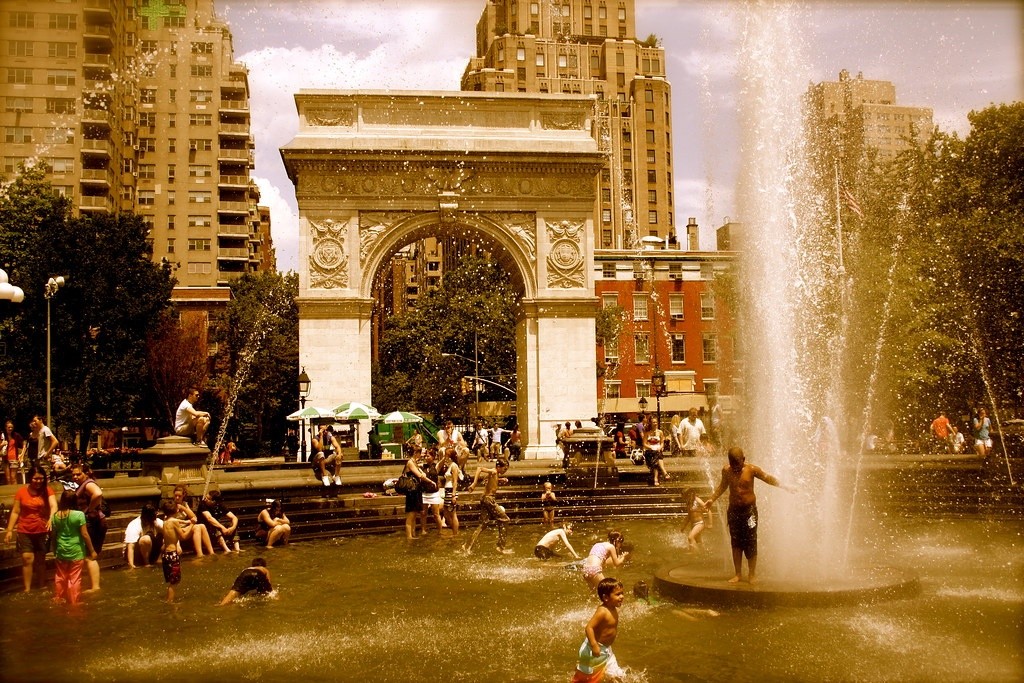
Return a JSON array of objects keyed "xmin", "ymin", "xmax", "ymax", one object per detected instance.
[{"xmin": 395, "ymin": 462, "xmax": 416, "ymax": 494}]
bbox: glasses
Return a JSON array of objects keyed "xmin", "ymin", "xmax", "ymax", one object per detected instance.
[{"xmin": 190, "ymin": 394, "xmax": 199, "ymax": 397}]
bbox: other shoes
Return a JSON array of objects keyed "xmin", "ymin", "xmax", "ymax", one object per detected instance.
[
  {"xmin": 654, "ymin": 481, "xmax": 661, "ymax": 486},
  {"xmin": 193, "ymin": 440, "xmax": 208, "ymax": 448},
  {"xmin": 664, "ymin": 475, "xmax": 671, "ymax": 479}
]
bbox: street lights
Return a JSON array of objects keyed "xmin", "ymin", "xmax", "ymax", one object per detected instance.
[
  {"xmin": 43, "ymin": 276, "xmax": 65, "ymax": 429},
  {"xmin": 705, "ymin": 388, "xmax": 715, "ymax": 445},
  {"xmin": 296, "ymin": 365, "xmax": 312, "ymax": 462},
  {"xmin": 651, "ymin": 363, "xmax": 667, "ymax": 430},
  {"xmin": 441, "ymin": 327, "xmax": 478, "ymax": 418}
]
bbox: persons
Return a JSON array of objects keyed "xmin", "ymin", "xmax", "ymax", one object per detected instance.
[
  {"xmin": 570, "ymin": 578, "xmax": 624, "ymax": 683},
  {"xmin": 866, "ymin": 433, "xmax": 879, "ymax": 449},
  {"xmin": 282, "ymin": 428, "xmax": 300, "ymax": 462},
  {"xmin": 632, "ymin": 579, "xmax": 656, "ymax": 606},
  {"xmin": 541, "ymin": 481, "xmax": 557, "ymax": 526},
  {"xmin": 461, "ymin": 457, "xmax": 509, "ymax": 554},
  {"xmin": 581, "ymin": 530, "xmax": 630, "ymax": 590},
  {"xmin": 555, "ymin": 396, "xmax": 723, "ymax": 487},
  {"xmin": 930, "ymin": 406, "xmax": 994, "ymax": 456},
  {"xmin": 0, "ymin": 415, "xmax": 108, "ymax": 604},
  {"xmin": 255, "ymin": 499, "xmax": 291, "ymax": 546},
  {"xmin": 308, "ymin": 421, "xmax": 343, "ymax": 486},
  {"xmin": 122, "ymin": 485, "xmax": 240, "ymax": 603},
  {"xmin": 401, "ymin": 420, "xmax": 469, "ymax": 538},
  {"xmin": 681, "ymin": 486, "xmax": 713, "ymax": 543},
  {"xmin": 367, "ymin": 430, "xmax": 382, "ymax": 459},
  {"xmin": 702, "ymin": 446, "xmax": 796, "ymax": 586},
  {"xmin": 215, "ymin": 432, "xmax": 236, "ymax": 466},
  {"xmin": 174, "ymin": 388, "xmax": 211, "ymax": 448},
  {"xmin": 219, "ymin": 558, "xmax": 273, "ymax": 604},
  {"xmin": 534, "ymin": 521, "xmax": 579, "ymax": 560},
  {"xmin": 472, "ymin": 421, "xmax": 523, "ymax": 462}
]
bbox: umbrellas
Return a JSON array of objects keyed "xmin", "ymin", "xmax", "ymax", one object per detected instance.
[
  {"xmin": 333, "ymin": 401, "xmax": 384, "ymax": 447},
  {"xmin": 373, "ymin": 409, "xmax": 424, "ymax": 445},
  {"xmin": 286, "ymin": 405, "xmax": 335, "ymax": 438}
]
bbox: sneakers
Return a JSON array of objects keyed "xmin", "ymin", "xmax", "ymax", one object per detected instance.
[
  {"xmin": 322, "ymin": 475, "xmax": 330, "ymax": 486},
  {"xmin": 332, "ymin": 475, "xmax": 342, "ymax": 485}
]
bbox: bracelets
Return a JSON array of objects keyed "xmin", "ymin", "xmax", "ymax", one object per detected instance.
[{"xmin": 452, "ymin": 496, "xmax": 455, "ymax": 497}]
